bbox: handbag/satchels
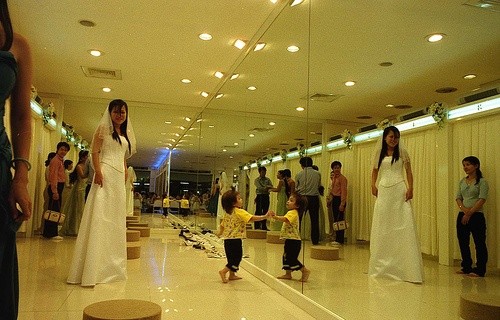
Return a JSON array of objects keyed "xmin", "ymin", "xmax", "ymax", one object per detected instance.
[
  {"xmin": 333, "ymin": 210, "xmax": 350, "ymax": 232},
  {"xmin": 43, "ymin": 198, "xmax": 66, "ymax": 224}
]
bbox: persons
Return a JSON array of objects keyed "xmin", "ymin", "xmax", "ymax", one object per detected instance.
[
  {"xmin": 126, "ymin": 166, "xmax": 136, "ymax": 217},
  {"xmin": 163, "ymin": 194, "xmax": 174, "ymax": 219},
  {"xmin": 59, "ymin": 160, "xmax": 73, "ymax": 225},
  {"xmin": 43, "ymin": 141, "xmax": 70, "ymax": 237},
  {"xmin": 273, "ymin": 192, "xmax": 310, "ymax": 282},
  {"xmin": 331, "ymin": 161, "xmax": 347, "ymax": 245},
  {"xmin": 301, "ymin": 165, "xmax": 326, "ymax": 242},
  {"xmin": 283, "ymin": 170, "xmax": 295, "ymax": 194},
  {"xmin": 361, "ymin": 126, "xmax": 425, "ymax": 284},
  {"xmin": 67, "ymin": 99, "xmax": 136, "ymax": 287},
  {"xmin": 217, "ymin": 172, "xmax": 232, "ymax": 219},
  {"xmin": 170, "ymin": 177, "xmax": 220, "ymax": 216},
  {"xmin": 295, "ymin": 157, "xmax": 320, "ymax": 246},
  {"xmin": 254, "ymin": 166, "xmax": 275, "ymax": 231},
  {"xmin": 0, "ymin": 0, "xmax": 31, "ymax": 320},
  {"xmin": 133, "ymin": 190, "xmax": 161, "ymax": 213},
  {"xmin": 266, "ymin": 169, "xmax": 291, "ymax": 231},
  {"xmin": 174, "ymin": 193, "xmax": 190, "ymax": 218},
  {"xmin": 326, "ymin": 170, "xmax": 336, "ymax": 240},
  {"xmin": 58, "ymin": 150, "xmax": 91, "ymax": 237},
  {"xmin": 456, "ymin": 156, "xmax": 489, "ymax": 278},
  {"xmin": 40, "ymin": 151, "xmax": 56, "ymax": 235},
  {"xmin": 216, "ymin": 189, "xmax": 272, "ymax": 283}
]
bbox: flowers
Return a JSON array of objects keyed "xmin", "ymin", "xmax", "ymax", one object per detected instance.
[
  {"xmin": 428, "ymin": 101, "xmax": 449, "ymax": 130},
  {"xmin": 296, "ymin": 144, "xmax": 305, "ymax": 157},
  {"xmin": 256, "ymin": 158, "xmax": 261, "ymax": 166},
  {"xmin": 43, "ymin": 102, "xmax": 55, "ymax": 125},
  {"xmin": 30, "ymin": 85, "xmax": 37, "ymax": 102},
  {"xmin": 266, "ymin": 152, "xmax": 273, "ymax": 163},
  {"xmin": 246, "ymin": 161, "xmax": 251, "ymax": 169},
  {"xmin": 67, "ymin": 125, "xmax": 89, "ymax": 149},
  {"xmin": 340, "ymin": 128, "xmax": 355, "ymax": 150},
  {"xmin": 281, "ymin": 149, "xmax": 288, "ymax": 163},
  {"xmin": 376, "ymin": 119, "xmax": 392, "ymax": 131}
]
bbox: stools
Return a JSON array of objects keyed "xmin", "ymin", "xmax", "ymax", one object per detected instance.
[
  {"xmin": 460, "ymin": 294, "xmax": 500, "ymax": 320},
  {"xmin": 199, "ymin": 213, "xmax": 211, "ymax": 217},
  {"xmin": 83, "ymin": 299, "xmax": 162, "ymax": 320},
  {"xmin": 246, "ymin": 229, "xmax": 285, "ymax": 243},
  {"xmin": 310, "ymin": 246, "xmax": 339, "ymax": 260},
  {"xmin": 126, "ymin": 216, "xmax": 150, "ymax": 259}
]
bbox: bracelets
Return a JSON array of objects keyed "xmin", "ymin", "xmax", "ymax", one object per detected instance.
[
  {"xmin": 9, "ymin": 158, "xmax": 31, "ymax": 171},
  {"xmin": 459, "ymin": 205, "xmax": 464, "ymax": 209}
]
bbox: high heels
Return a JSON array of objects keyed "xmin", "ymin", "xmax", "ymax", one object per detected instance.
[{"xmin": 179, "ymin": 238, "xmax": 192, "ymax": 246}]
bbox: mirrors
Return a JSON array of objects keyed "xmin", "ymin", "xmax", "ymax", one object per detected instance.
[{"xmin": 169, "ymin": 0, "xmax": 500, "ymax": 320}]
